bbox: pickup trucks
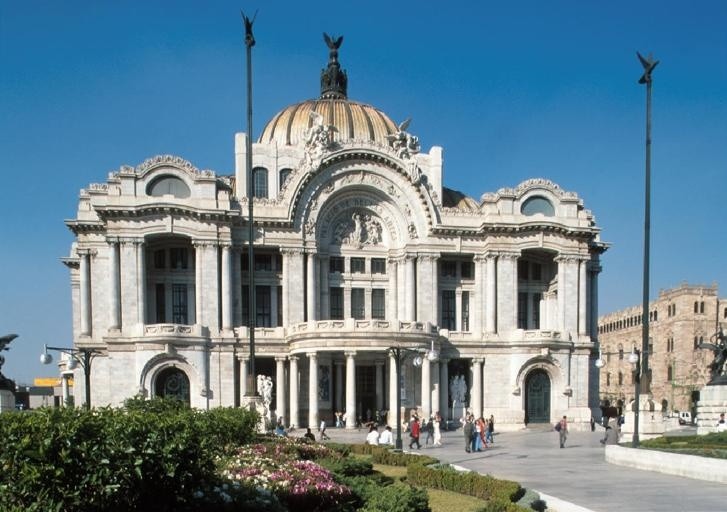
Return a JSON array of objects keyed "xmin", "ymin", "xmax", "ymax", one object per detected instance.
[{"xmin": 670, "ymin": 412, "xmax": 698, "ymax": 424}]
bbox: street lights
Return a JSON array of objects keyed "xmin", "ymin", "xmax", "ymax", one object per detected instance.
[
  {"xmin": 384, "ymin": 343, "xmax": 438, "ymax": 454},
  {"xmin": 39, "ymin": 342, "xmax": 109, "ymax": 411},
  {"xmin": 630, "ymin": 343, "xmax": 643, "ymax": 445}
]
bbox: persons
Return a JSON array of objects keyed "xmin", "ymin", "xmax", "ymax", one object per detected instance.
[
  {"xmin": 351, "ymin": 212, "xmax": 363, "ymax": 243},
  {"xmin": 276, "ymin": 408, "xmax": 494, "ymax": 452},
  {"xmin": 558, "ymin": 415, "xmax": 567, "ymax": 448},
  {"xmin": 450, "ymin": 375, "xmax": 458, "ymax": 400},
  {"xmin": 260, "ymin": 375, "xmax": 269, "ymax": 395},
  {"xmin": 718, "ymin": 413, "xmax": 724, "ymax": 423},
  {"xmin": 265, "ymin": 375, "xmax": 272, "ymax": 402},
  {"xmin": 598, "ymin": 425, "xmax": 619, "ymax": 445},
  {"xmin": 458, "ymin": 375, "xmax": 466, "ymax": 402},
  {"xmin": 590, "ymin": 416, "xmax": 594, "ymax": 431}
]
